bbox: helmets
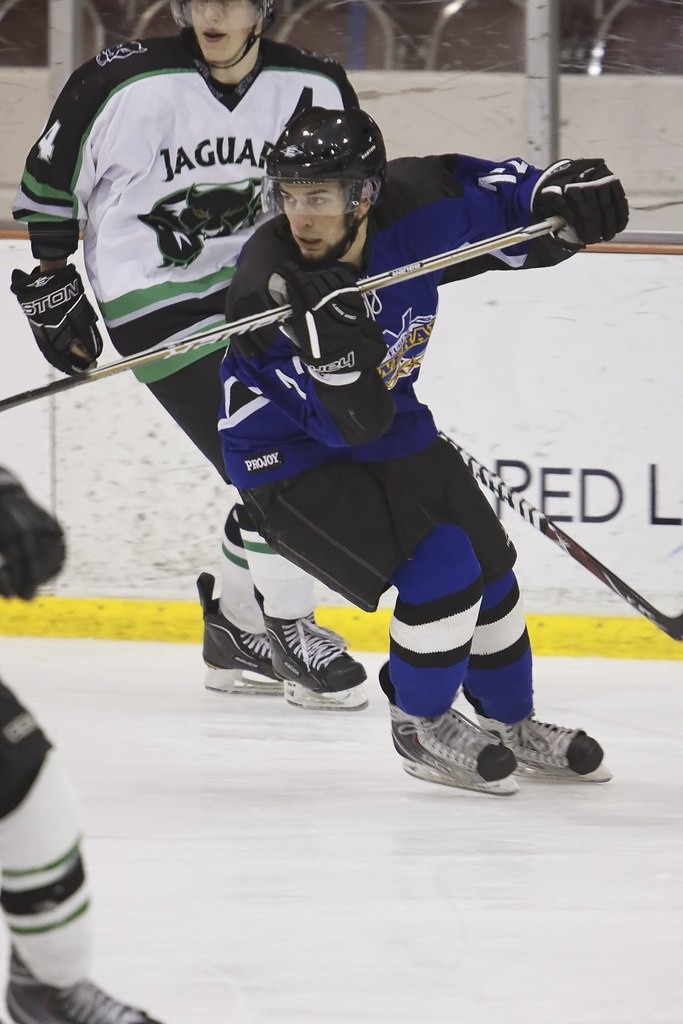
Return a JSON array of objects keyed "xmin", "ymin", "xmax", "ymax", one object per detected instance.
[{"xmin": 266, "ymin": 106, "xmax": 387, "ymax": 210}]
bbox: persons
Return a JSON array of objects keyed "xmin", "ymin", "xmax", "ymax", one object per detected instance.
[
  {"xmin": 0, "ymin": 468, "xmax": 162, "ymax": 1024},
  {"xmin": 11, "ymin": 0, "xmax": 367, "ymax": 709},
  {"xmin": 218, "ymin": 106, "xmax": 628, "ymax": 796}
]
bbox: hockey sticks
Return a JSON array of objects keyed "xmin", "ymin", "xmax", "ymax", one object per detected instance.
[
  {"xmin": 0, "ymin": 214, "xmax": 571, "ymax": 415},
  {"xmin": 434, "ymin": 423, "xmax": 683, "ymax": 643}
]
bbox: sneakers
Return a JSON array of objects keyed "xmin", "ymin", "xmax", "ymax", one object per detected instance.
[
  {"xmin": 196, "ymin": 571, "xmax": 369, "ymax": 711},
  {"xmin": 388, "ymin": 700, "xmax": 613, "ymax": 795},
  {"xmin": 6, "ymin": 948, "xmax": 164, "ymax": 1024}
]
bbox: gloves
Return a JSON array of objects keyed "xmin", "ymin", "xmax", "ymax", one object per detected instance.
[
  {"xmin": 11, "ymin": 265, "xmax": 103, "ymax": 376},
  {"xmin": 532, "ymin": 158, "xmax": 630, "ymax": 252},
  {"xmin": 0, "ymin": 467, "xmax": 67, "ymax": 600},
  {"xmin": 287, "ymin": 268, "xmax": 397, "ymax": 447}
]
